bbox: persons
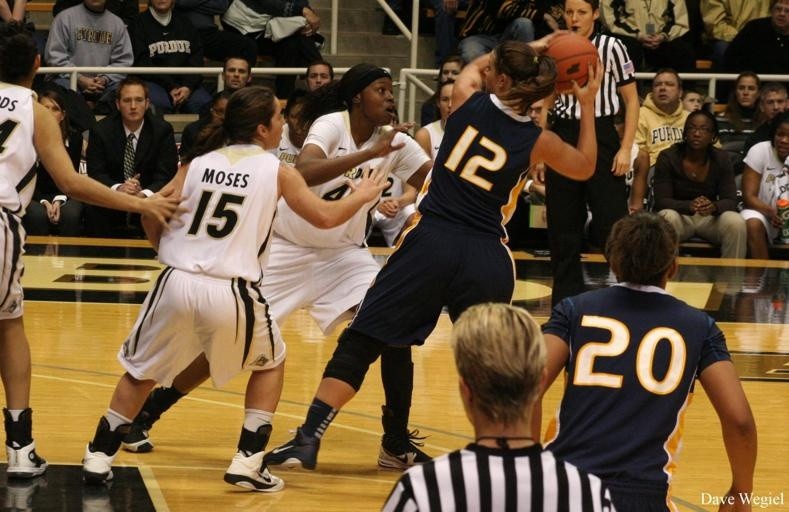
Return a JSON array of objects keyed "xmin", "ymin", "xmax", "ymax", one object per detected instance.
[
  {"xmin": 118, "ymin": 61, "xmax": 437, "ymax": 471},
  {"xmin": 77, "ymin": 84, "xmax": 392, "ymax": 494},
  {"xmin": 385, "ymin": 300, "xmax": 623, "ymax": 512},
  {"xmin": 0, "ymin": 0, "xmax": 789, "ymax": 321},
  {"xmin": 532, "ymin": 208, "xmax": 758, "ymax": 512},
  {"xmin": 263, "ymin": 27, "xmax": 608, "ymax": 472},
  {"xmin": 0, "ymin": 16, "xmax": 193, "ymax": 488}
]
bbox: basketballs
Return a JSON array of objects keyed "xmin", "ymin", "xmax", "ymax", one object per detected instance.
[{"xmin": 542, "ymin": 33, "xmax": 599, "ymax": 93}]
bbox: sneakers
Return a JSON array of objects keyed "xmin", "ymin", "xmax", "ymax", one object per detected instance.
[
  {"xmin": 379, "ymin": 434, "xmax": 432, "ymax": 469},
  {"xmin": 6, "ymin": 441, "xmax": 48, "ymax": 478},
  {"xmin": 224, "ymin": 450, "xmax": 285, "ymax": 493},
  {"xmin": 265, "ymin": 427, "xmax": 319, "ymax": 470},
  {"xmin": 82, "ymin": 441, "xmax": 115, "ymax": 482},
  {"xmin": 122, "ymin": 423, "xmax": 153, "ymax": 452}
]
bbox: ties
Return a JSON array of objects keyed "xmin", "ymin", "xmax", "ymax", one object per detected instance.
[{"xmin": 124, "ymin": 134, "xmax": 137, "ymax": 180}]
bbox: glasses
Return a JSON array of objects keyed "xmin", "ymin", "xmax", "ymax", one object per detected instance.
[{"xmin": 687, "ymin": 125, "xmax": 710, "ymax": 134}]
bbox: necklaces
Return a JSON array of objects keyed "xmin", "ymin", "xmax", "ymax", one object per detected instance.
[{"xmin": 477, "ymin": 432, "xmax": 534, "ymax": 450}]
